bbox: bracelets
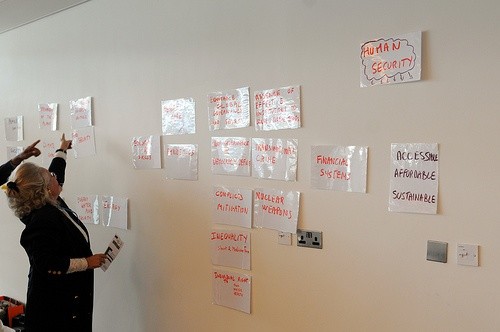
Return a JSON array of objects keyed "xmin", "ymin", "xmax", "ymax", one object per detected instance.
[{"xmin": 56, "ymin": 148, "xmax": 67, "ymax": 154}]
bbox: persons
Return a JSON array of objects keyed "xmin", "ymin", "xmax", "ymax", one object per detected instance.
[
  {"xmin": 5, "ymin": 133, "xmax": 107, "ymax": 332},
  {"xmin": 0, "ymin": 139, "xmax": 41, "ymax": 187}
]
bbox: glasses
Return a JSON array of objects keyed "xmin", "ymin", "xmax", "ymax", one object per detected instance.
[
  {"xmin": 64, "ymin": 207, "xmax": 78, "ymax": 219},
  {"xmin": 50, "ymin": 171, "xmax": 56, "ymax": 177}
]
